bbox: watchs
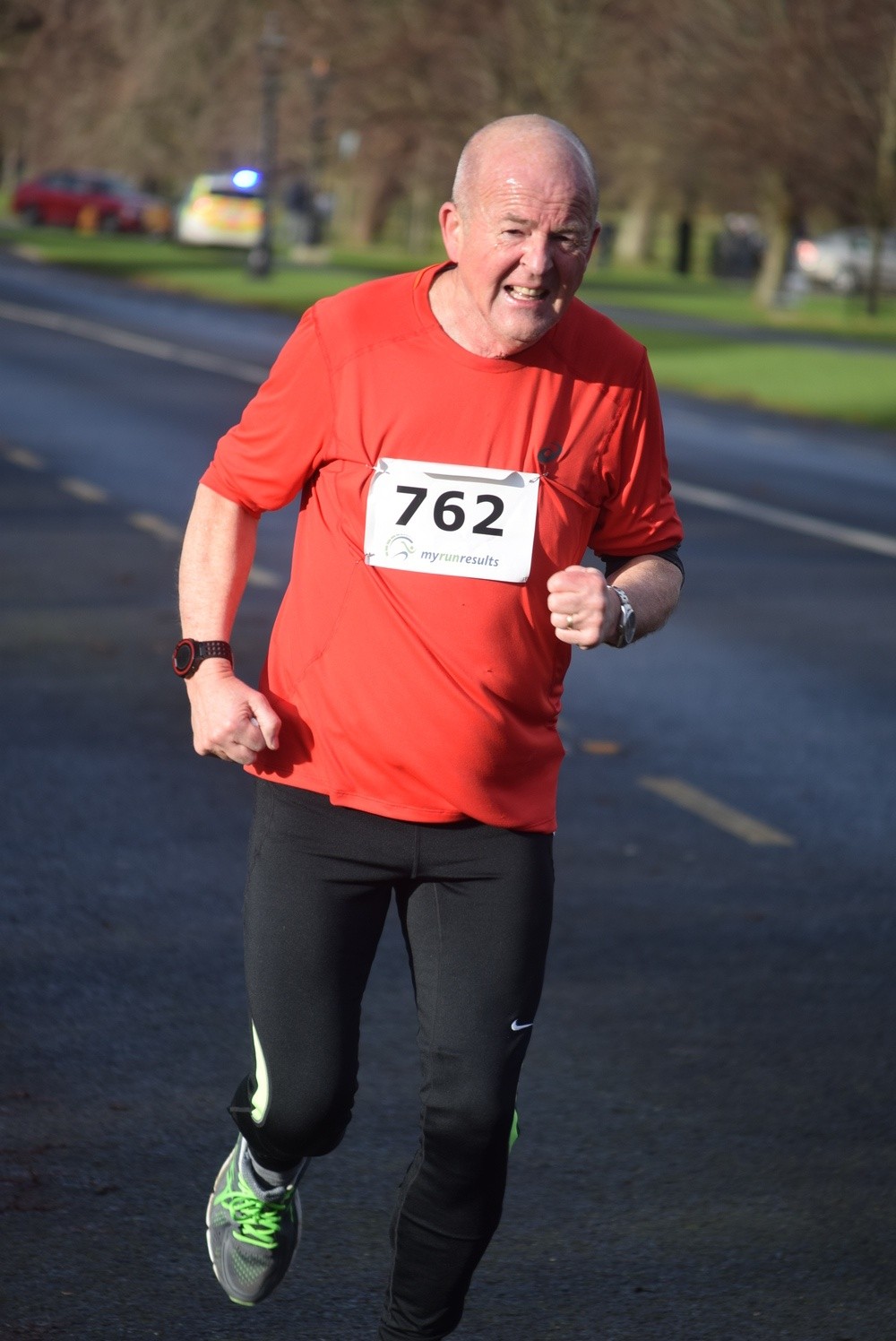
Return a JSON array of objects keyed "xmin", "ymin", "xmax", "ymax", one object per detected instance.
[
  {"xmin": 172, "ymin": 639, "xmax": 234, "ymax": 677},
  {"xmin": 604, "ymin": 585, "xmax": 636, "ymax": 648}
]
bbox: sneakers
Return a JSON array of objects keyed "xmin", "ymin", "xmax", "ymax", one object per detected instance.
[{"xmin": 205, "ymin": 1131, "xmax": 302, "ymax": 1308}]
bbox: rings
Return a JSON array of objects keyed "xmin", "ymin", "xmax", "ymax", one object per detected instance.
[{"xmin": 567, "ymin": 614, "xmax": 573, "ymax": 630}]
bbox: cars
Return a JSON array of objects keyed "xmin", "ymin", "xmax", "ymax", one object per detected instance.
[
  {"xmin": 12, "ymin": 168, "xmax": 159, "ymax": 240},
  {"xmin": 174, "ymin": 173, "xmax": 312, "ymax": 256},
  {"xmin": 798, "ymin": 225, "xmax": 895, "ymax": 299}
]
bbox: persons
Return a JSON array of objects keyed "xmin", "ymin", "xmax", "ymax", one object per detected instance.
[{"xmin": 172, "ymin": 114, "xmax": 687, "ymax": 1341}]
